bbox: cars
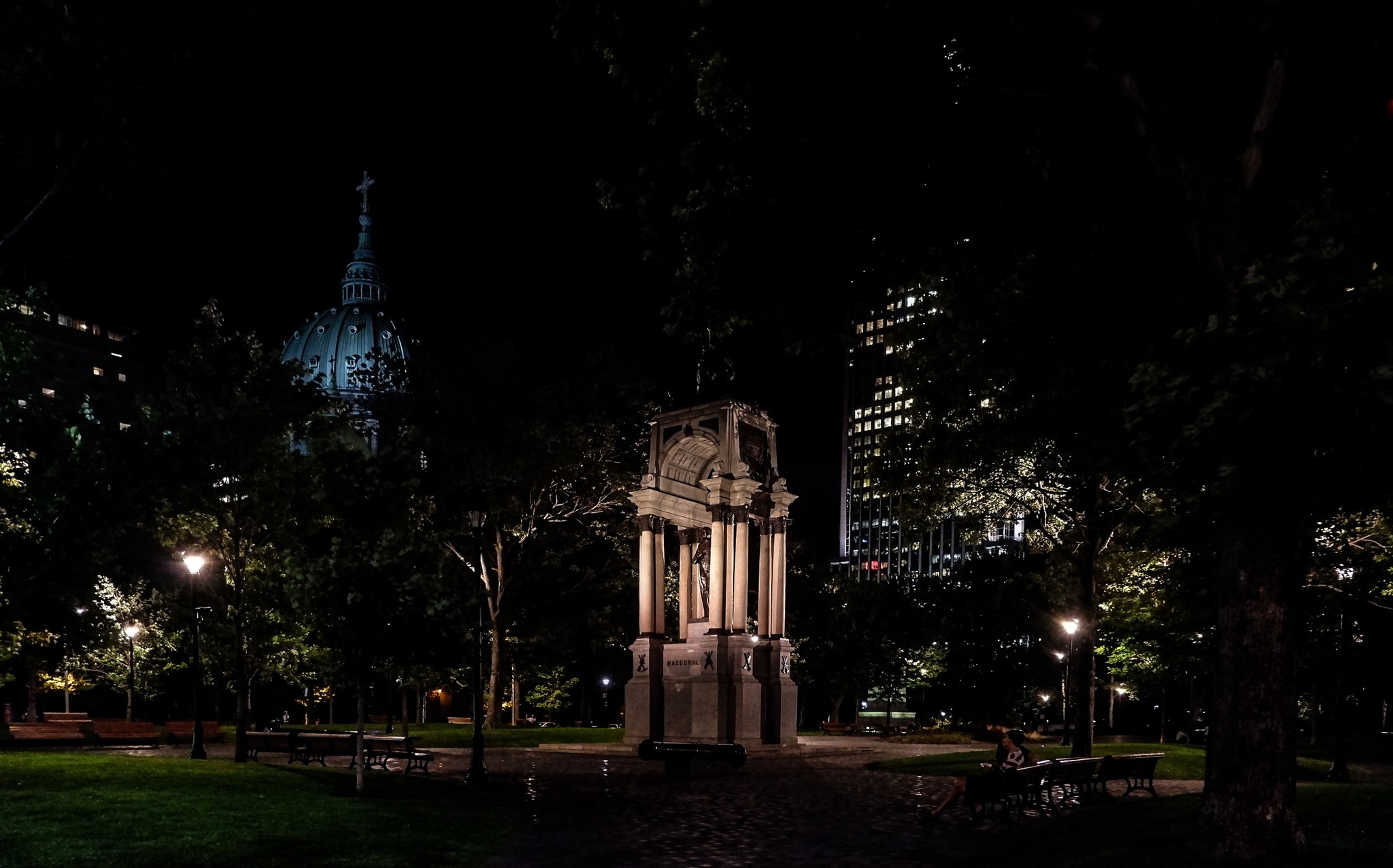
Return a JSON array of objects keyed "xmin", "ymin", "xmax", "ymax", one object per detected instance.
[
  {"xmin": 607, "ymin": 724, "xmax": 623, "ymax": 729},
  {"xmin": 539, "ymin": 722, "xmax": 562, "ymax": 728}
]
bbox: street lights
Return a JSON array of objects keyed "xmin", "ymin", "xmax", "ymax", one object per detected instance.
[
  {"xmin": 177, "ymin": 547, "xmax": 215, "ymax": 759},
  {"xmin": 121, "ymin": 619, "xmax": 146, "ymax": 722},
  {"xmin": 1060, "ymin": 620, "xmax": 1078, "ymax": 746}
]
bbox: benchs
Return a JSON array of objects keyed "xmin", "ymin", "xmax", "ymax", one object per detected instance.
[
  {"xmin": 964, "ymin": 752, "xmax": 1167, "ymax": 824},
  {"xmin": 243, "ymin": 731, "xmax": 434, "ymax": 777},
  {"xmin": 822, "ymin": 721, "xmax": 896, "ymax": 737},
  {"xmin": 3, "ymin": 712, "xmax": 227, "ymax": 750}
]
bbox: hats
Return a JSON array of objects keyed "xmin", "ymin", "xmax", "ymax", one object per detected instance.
[{"xmin": 1006, "ymin": 730, "xmax": 1025, "ymax": 747}]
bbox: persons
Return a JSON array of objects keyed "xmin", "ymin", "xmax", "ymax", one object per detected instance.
[
  {"xmin": 691, "ymin": 526, "xmax": 711, "ymax": 618},
  {"xmin": 915, "ymin": 730, "xmax": 1037, "ymax": 826},
  {"xmin": 282, "ymin": 711, "xmax": 289, "ymax": 725}
]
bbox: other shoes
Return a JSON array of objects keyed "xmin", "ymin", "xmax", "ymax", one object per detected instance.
[{"xmin": 916, "ymin": 809, "xmax": 940, "ymax": 820}]
